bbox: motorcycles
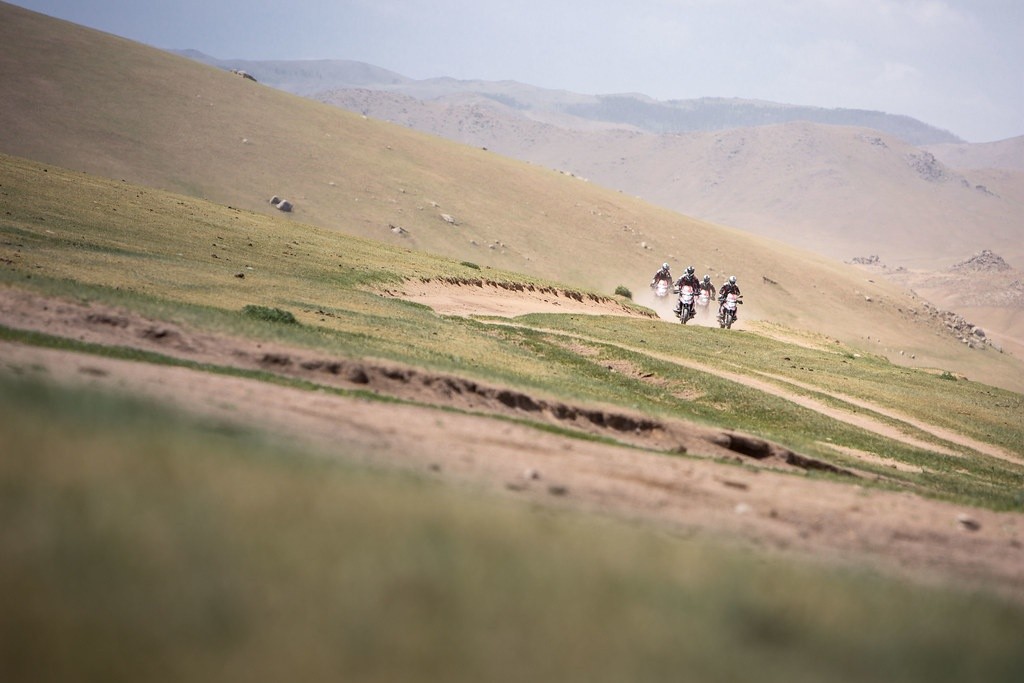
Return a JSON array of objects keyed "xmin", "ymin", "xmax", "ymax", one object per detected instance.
[{"xmin": 650, "ymin": 279, "xmax": 744, "ymax": 330}]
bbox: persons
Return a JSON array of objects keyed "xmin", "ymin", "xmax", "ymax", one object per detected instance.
[
  {"xmin": 716, "ymin": 276, "xmax": 743, "ymax": 323},
  {"xmin": 699, "ymin": 275, "xmax": 716, "ymax": 301},
  {"xmin": 649, "ymin": 263, "xmax": 673, "ymax": 287},
  {"xmin": 674, "ymin": 265, "xmax": 701, "ymax": 318}
]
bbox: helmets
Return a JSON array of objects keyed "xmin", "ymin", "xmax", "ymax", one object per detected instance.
[
  {"xmin": 704, "ymin": 275, "xmax": 710, "ymax": 283},
  {"xmin": 730, "ymin": 275, "xmax": 737, "ymax": 287},
  {"xmin": 661, "ymin": 262, "xmax": 670, "ymax": 271},
  {"xmin": 687, "ymin": 265, "xmax": 695, "ymax": 274}
]
